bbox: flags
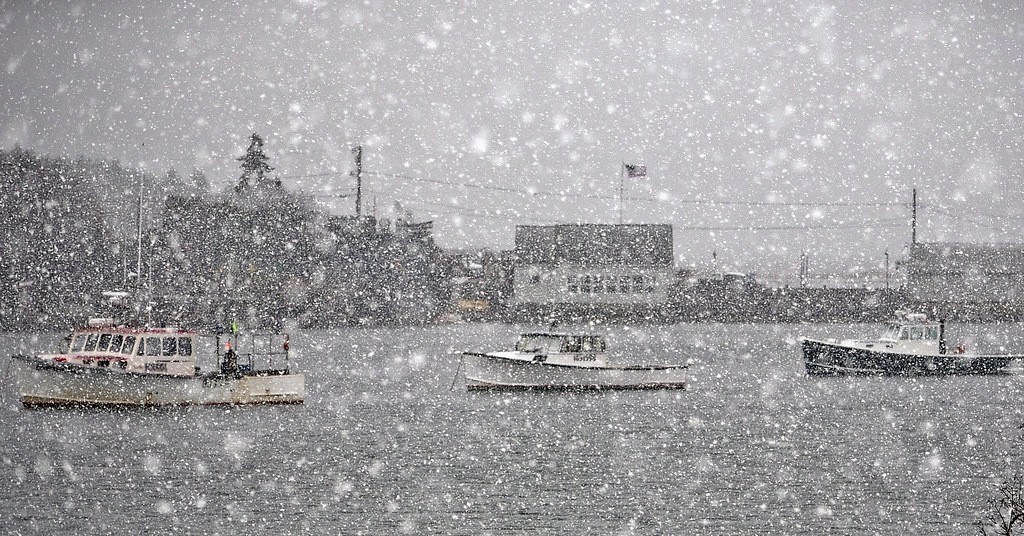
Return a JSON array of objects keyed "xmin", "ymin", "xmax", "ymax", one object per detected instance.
[{"xmin": 625, "ymin": 164, "xmax": 646, "ymax": 177}]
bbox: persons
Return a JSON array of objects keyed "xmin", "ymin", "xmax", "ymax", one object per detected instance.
[
  {"xmin": 924, "ymin": 318, "xmax": 932, "ymax": 325},
  {"xmin": 219, "ymin": 337, "xmax": 240, "ymax": 374},
  {"xmin": 958, "ymin": 344, "xmax": 965, "ymax": 356},
  {"xmin": 560, "ymin": 339, "xmax": 580, "ymax": 353},
  {"xmin": 531, "ymin": 349, "xmax": 547, "ymax": 367},
  {"xmin": 82, "ymin": 356, "xmax": 127, "ymax": 369},
  {"xmin": 548, "ymin": 309, "xmax": 562, "ymax": 331}
]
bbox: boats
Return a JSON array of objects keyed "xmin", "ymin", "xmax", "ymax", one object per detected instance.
[
  {"xmin": 800, "ymin": 308, "xmax": 1018, "ymax": 376},
  {"xmin": 457, "ymin": 331, "xmax": 691, "ymax": 391},
  {"xmin": 10, "ymin": 288, "xmax": 307, "ymax": 405}
]
